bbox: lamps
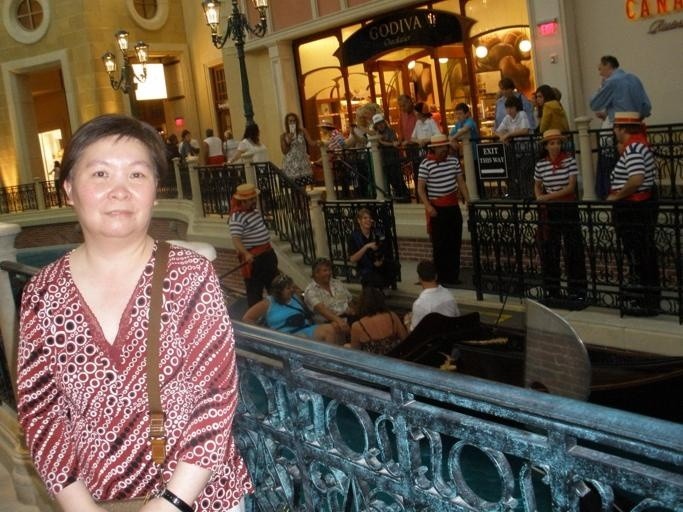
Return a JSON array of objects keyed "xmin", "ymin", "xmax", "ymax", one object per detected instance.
[{"xmin": 536, "ymin": 16, "xmax": 557, "ymax": 36}]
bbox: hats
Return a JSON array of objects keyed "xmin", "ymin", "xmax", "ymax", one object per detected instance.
[
  {"xmin": 540, "ymin": 129, "xmax": 567, "ymax": 144},
  {"xmin": 316, "ymin": 117, "xmax": 336, "ymax": 128},
  {"xmin": 610, "ymin": 112, "xmax": 646, "ymax": 124},
  {"xmin": 233, "ymin": 184, "xmax": 261, "ymax": 200},
  {"xmin": 427, "ymin": 135, "xmax": 451, "ymax": 147},
  {"xmin": 372, "ymin": 114, "xmax": 384, "ymax": 124},
  {"xmin": 414, "ymin": 103, "xmax": 429, "ymax": 115}
]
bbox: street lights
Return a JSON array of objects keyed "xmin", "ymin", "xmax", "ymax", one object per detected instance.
[
  {"xmin": 200, "ymin": 1, "xmax": 268, "ymax": 129},
  {"xmin": 100, "ymin": 30, "xmax": 149, "ymax": 120}
]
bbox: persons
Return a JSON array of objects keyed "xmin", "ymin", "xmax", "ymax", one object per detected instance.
[
  {"xmin": 49, "ymin": 161, "xmax": 62, "ymax": 208},
  {"xmin": 227, "ymin": 183, "xmax": 278, "ymax": 309},
  {"xmin": 606, "ymin": 110, "xmax": 662, "ymax": 308},
  {"xmin": 169, "ymin": 122, "xmax": 270, "ymax": 187},
  {"xmin": 279, "ymin": 113, "xmax": 323, "ymax": 224},
  {"xmin": 240, "ymin": 257, "xmax": 462, "ymax": 356},
  {"xmin": 532, "ymin": 127, "xmax": 588, "ymax": 296},
  {"xmin": 318, "ymin": 77, "xmax": 574, "ymax": 188},
  {"xmin": 416, "ymin": 133, "xmax": 471, "ymax": 286},
  {"xmin": 590, "ymin": 55, "xmax": 653, "ymax": 123},
  {"xmin": 15, "ymin": 113, "xmax": 257, "ymax": 512},
  {"xmin": 347, "ymin": 209, "xmax": 387, "ymax": 277}
]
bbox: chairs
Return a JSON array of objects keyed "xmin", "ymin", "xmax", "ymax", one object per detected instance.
[{"xmin": 256, "ymin": 290, "xmax": 482, "ymax": 383}]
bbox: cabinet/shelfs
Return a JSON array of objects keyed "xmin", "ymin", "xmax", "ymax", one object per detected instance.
[{"xmin": 306, "ymin": 92, "xmax": 399, "ymax": 182}]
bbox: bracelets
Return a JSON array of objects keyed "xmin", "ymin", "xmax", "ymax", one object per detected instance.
[{"xmin": 160, "ymin": 488, "xmax": 194, "ymax": 512}]
